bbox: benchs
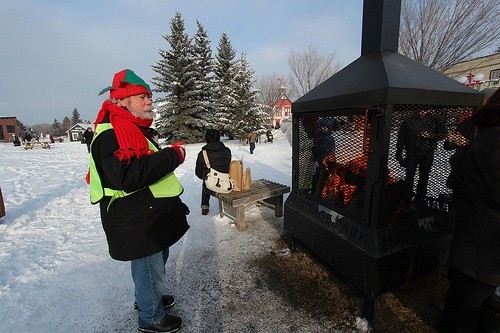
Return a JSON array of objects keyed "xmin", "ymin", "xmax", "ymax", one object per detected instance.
[{"xmin": 214, "ymin": 178, "xmax": 290, "ymax": 231}]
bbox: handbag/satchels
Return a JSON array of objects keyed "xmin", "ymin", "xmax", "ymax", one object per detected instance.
[{"xmin": 204, "ymin": 167, "xmax": 234, "ymax": 193}]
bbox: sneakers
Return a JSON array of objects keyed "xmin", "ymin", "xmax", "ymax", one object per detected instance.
[
  {"xmin": 136, "ymin": 313, "xmax": 183, "ymax": 333},
  {"xmin": 202, "ymin": 209, "xmax": 209, "ymax": 215},
  {"xmin": 134, "ymin": 294, "xmax": 175, "ymax": 308}
]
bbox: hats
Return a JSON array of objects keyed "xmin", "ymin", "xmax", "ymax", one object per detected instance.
[{"xmin": 97, "ymin": 69, "xmax": 153, "ymax": 99}]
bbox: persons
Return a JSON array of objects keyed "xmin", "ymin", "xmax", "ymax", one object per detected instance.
[
  {"xmin": 280, "ymin": 116, "xmax": 335, "ymax": 201},
  {"xmin": 82, "ymin": 127, "xmax": 94, "ymax": 153},
  {"xmin": 89, "ymin": 69, "xmax": 190, "ymax": 333},
  {"xmin": 247, "ymin": 129, "xmax": 258, "ymax": 154},
  {"xmin": 396, "ymin": 109, "xmax": 447, "ymax": 196},
  {"xmin": 195, "ymin": 129, "xmax": 232, "ymax": 215},
  {"xmin": 266, "ymin": 129, "xmax": 274, "ymax": 142},
  {"xmin": 441, "ymin": 90, "xmax": 500, "ymax": 333}
]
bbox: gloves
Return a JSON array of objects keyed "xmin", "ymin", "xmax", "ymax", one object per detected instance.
[{"xmin": 171, "ymin": 146, "xmax": 186, "ymax": 164}]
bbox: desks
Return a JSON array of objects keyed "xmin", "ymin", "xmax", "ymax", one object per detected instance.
[{"xmin": 25, "ymin": 141, "xmax": 50, "ymax": 149}]
